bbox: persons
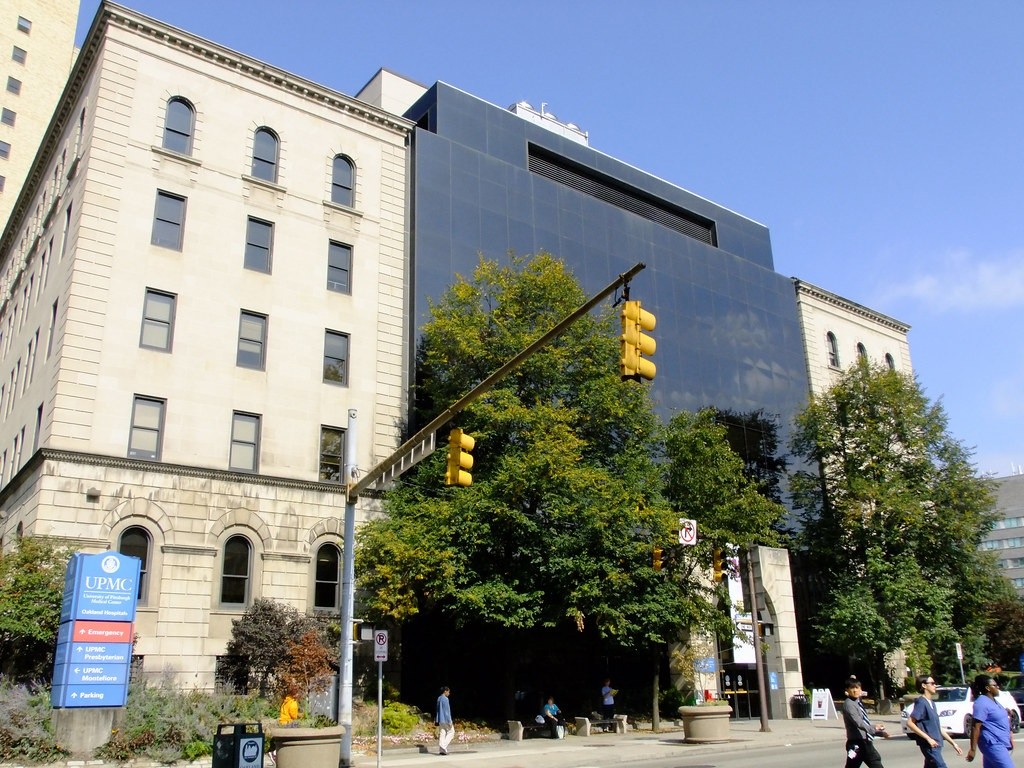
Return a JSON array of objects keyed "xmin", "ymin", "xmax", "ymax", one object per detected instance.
[
  {"xmin": 966, "ymin": 675, "xmax": 1015, "ymax": 768},
  {"xmin": 845, "ymin": 675, "xmax": 867, "ymax": 700},
  {"xmin": 543, "ymin": 697, "xmax": 565, "ymax": 738},
  {"xmin": 436, "ymin": 686, "xmax": 455, "ymax": 754},
  {"xmin": 602, "ymin": 679, "xmax": 614, "ymax": 719},
  {"xmin": 268, "ymin": 690, "xmax": 303, "ymax": 764},
  {"xmin": 843, "ymin": 678, "xmax": 890, "ymax": 768},
  {"xmin": 907, "ymin": 676, "xmax": 963, "ymax": 768}
]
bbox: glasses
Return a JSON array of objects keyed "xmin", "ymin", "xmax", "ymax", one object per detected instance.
[{"xmin": 924, "ymin": 681, "xmax": 936, "ymax": 685}]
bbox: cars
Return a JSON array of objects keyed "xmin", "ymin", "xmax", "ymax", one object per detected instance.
[
  {"xmin": 1004, "ymin": 675, "xmax": 1024, "ymax": 721},
  {"xmin": 899, "ymin": 684, "xmax": 1022, "ymax": 740}
]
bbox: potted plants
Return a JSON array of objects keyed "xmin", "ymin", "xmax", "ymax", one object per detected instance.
[
  {"xmin": 269, "ymin": 627, "xmax": 346, "ymax": 768},
  {"xmin": 678, "ymin": 699, "xmax": 733, "ymax": 744}
]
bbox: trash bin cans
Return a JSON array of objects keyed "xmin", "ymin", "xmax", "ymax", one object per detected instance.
[
  {"xmin": 793, "ymin": 694, "xmax": 810, "ymax": 718},
  {"xmin": 212, "ymin": 722, "xmax": 266, "ymax": 768}
]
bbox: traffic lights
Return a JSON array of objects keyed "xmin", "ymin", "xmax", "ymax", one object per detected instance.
[
  {"xmin": 444, "ymin": 428, "xmax": 475, "ymax": 487},
  {"xmin": 713, "ymin": 547, "xmax": 727, "ymax": 582},
  {"xmin": 619, "ymin": 300, "xmax": 657, "ymax": 381},
  {"xmin": 353, "ymin": 623, "xmax": 361, "ymax": 641},
  {"xmin": 654, "ymin": 546, "xmax": 665, "ymax": 572}
]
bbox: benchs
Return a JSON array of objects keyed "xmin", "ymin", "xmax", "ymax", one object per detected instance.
[
  {"xmin": 507, "ymin": 718, "xmax": 566, "ymax": 741},
  {"xmin": 575, "ymin": 714, "xmax": 627, "ymax": 737}
]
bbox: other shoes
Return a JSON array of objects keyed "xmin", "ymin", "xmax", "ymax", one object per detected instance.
[{"xmin": 439, "ymin": 747, "xmax": 447, "ymax": 755}]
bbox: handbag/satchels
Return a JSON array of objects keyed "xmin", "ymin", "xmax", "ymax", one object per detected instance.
[{"xmin": 535, "ymin": 715, "xmax": 545, "ymax": 724}]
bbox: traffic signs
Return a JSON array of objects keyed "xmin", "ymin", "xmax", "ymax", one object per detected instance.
[{"xmin": 64, "ymin": 621, "xmax": 130, "ymax": 708}]
